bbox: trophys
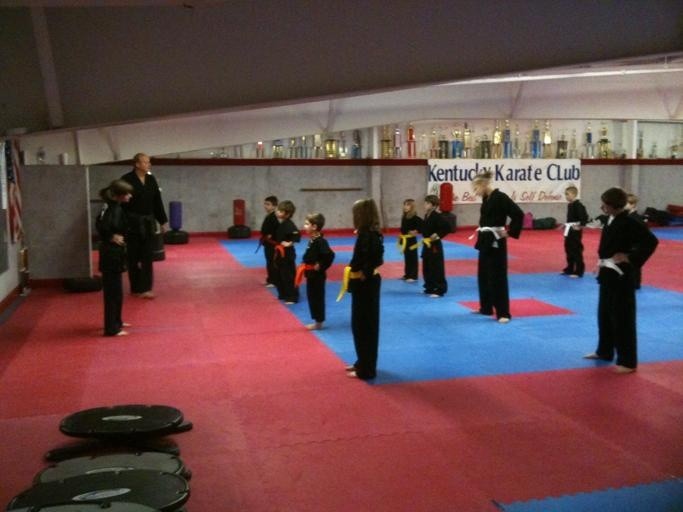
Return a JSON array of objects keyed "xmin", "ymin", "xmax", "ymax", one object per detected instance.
[
  {"xmin": 637, "ymin": 131, "xmax": 681, "ymax": 159},
  {"xmin": 214, "ymin": 131, "xmax": 368, "ymax": 161},
  {"xmin": 379, "ymin": 121, "xmax": 631, "ymax": 159}
]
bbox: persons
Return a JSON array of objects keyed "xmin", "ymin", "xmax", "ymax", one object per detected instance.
[
  {"xmin": 119, "ymin": 152, "xmax": 169, "ymax": 299},
  {"xmin": 96, "ymin": 179, "xmax": 135, "ymax": 337},
  {"xmin": 344, "ymin": 197, "xmax": 384, "ymax": 379},
  {"xmin": 558, "ymin": 185, "xmax": 589, "ymax": 279},
  {"xmin": 419, "ymin": 193, "xmax": 452, "ymax": 299},
  {"xmin": 300, "ymin": 212, "xmax": 335, "ymax": 330},
  {"xmin": 397, "ymin": 198, "xmax": 423, "ymax": 284},
  {"xmin": 582, "ymin": 186, "xmax": 659, "ymax": 375},
  {"xmin": 259, "ymin": 195, "xmax": 279, "ymax": 288},
  {"xmin": 625, "ymin": 193, "xmax": 642, "ymax": 290},
  {"xmin": 470, "ymin": 170, "xmax": 525, "ymax": 324},
  {"xmin": 272, "ymin": 199, "xmax": 301, "ymax": 305}
]
